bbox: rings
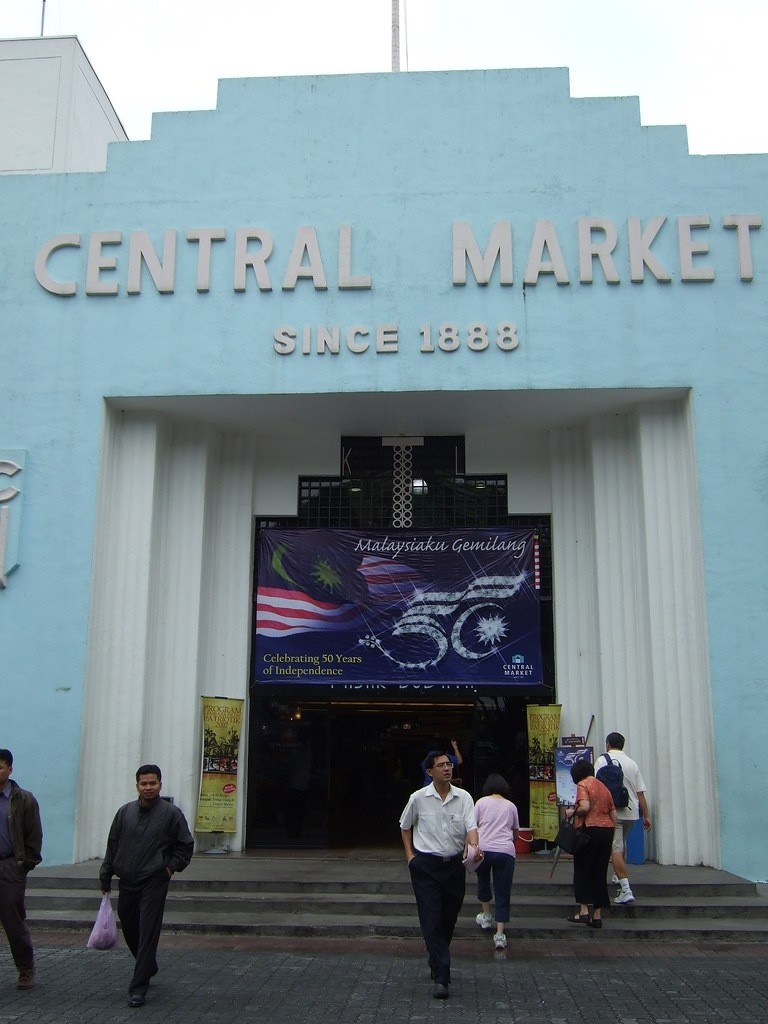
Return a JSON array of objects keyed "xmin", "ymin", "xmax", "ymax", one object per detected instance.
[{"xmin": 479, "ymin": 854, "xmax": 483, "ymax": 857}]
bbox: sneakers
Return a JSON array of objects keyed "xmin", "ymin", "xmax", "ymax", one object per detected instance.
[
  {"xmin": 494, "ymin": 934, "xmax": 507, "ymax": 949},
  {"xmin": 611, "ymin": 875, "xmax": 619, "ymax": 882},
  {"xmin": 475, "ymin": 912, "xmax": 495, "ymax": 929},
  {"xmin": 615, "ymin": 888, "xmax": 636, "ymax": 905},
  {"xmin": 15, "ymin": 961, "xmax": 36, "ymax": 989}
]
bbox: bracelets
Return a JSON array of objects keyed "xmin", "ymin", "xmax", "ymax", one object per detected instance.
[{"xmin": 471, "ymin": 844, "xmax": 477, "ymax": 847}]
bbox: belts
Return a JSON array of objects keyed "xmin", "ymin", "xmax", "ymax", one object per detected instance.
[{"xmin": 420, "ymin": 855, "xmax": 466, "ymax": 861}]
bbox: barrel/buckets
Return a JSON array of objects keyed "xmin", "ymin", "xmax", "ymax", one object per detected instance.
[{"xmin": 514, "ymin": 828, "xmax": 534, "ymax": 854}]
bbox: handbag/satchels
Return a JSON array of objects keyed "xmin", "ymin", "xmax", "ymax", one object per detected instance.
[
  {"xmin": 87, "ymin": 890, "xmax": 118, "ymax": 950},
  {"xmin": 554, "ymin": 806, "xmax": 582, "ymax": 854}
]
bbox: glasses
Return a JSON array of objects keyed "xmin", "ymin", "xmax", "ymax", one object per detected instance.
[{"xmin": 431, "ymin": 762, "xmax": 454, "ymax": 768}]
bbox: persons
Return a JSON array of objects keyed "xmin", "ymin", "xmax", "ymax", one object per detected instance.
[
  {"xmin": 565, "ymin": 760, "xmax": 618, "ymax": 929},
  {"xmin": 593, "ymin": 732, "xmax": 652, "ymax": 903},
  {"xmin": 399, "ymin": 752, "xmax": 485, "ymax": 998},
  {"xmin": 0, "ymin": 748, "xmax": 44, "ymax": 989},
  {"xmin": 463, "ymin": 774, "xmax": 519, "ymax": 949},
  {"xmin": 99, "ymin": 764, "xmax": 195, "ymax": 1007}
]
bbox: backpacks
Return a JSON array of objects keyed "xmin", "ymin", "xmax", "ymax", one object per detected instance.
[{"xmin": 595, "ymin": 753, "xmax": 629, "ymax": 808}]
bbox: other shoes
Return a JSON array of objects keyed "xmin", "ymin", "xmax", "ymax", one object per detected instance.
[
  {"xmin": 129, "ymin": 994, "xmax": 145, "ymax": 1007},
  {"xmin": 586, "ymin": 917, "xmax": 603, "ymax": 928},
  {"xmin": 431, "ymin": 969, "xmax": 435, "ymax": 979},
  {"xmin": 567, "ymin": 910, "xmax": 589, "ymax": 923},
  {"xmin": 433, "ymin": 983, "xmax": 449, "ymax": 999}
]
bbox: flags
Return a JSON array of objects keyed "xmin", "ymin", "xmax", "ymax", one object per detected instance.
[{"xmin": 257, "ymin": 555, "xmax": 429, "ymax": 639}]
